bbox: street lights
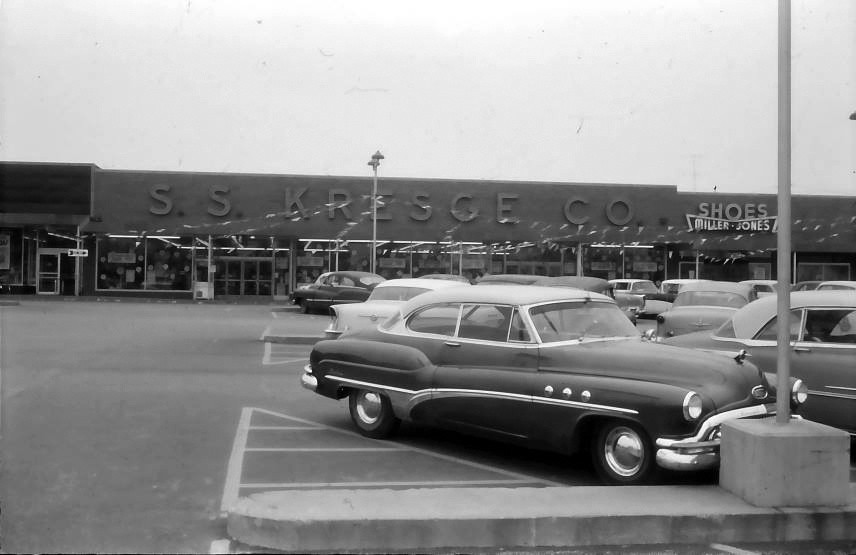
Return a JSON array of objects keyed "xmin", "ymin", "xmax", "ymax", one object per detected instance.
[{"xmin": 368, "ymin": 150, "xmax": 385, "ymax": 275}]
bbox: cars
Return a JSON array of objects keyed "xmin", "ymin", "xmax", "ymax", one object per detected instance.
[
  {"xmin": 301, "ymin": 287, "xmax": 811, "ymax": 487},
  {"xmin": 291, "ymin": 271, "xmax": 383, "ymax": 313},
  {"xmin": 419, "ymin": 274, "xmax": 856, "ymax": 347},
  {"xmin": 330, "ymin": 274, "xmax": 470, "ymax": 333},
  {"xmin": 645, "ymin": 290, "xmax": 856, "ymax": 438}
]
